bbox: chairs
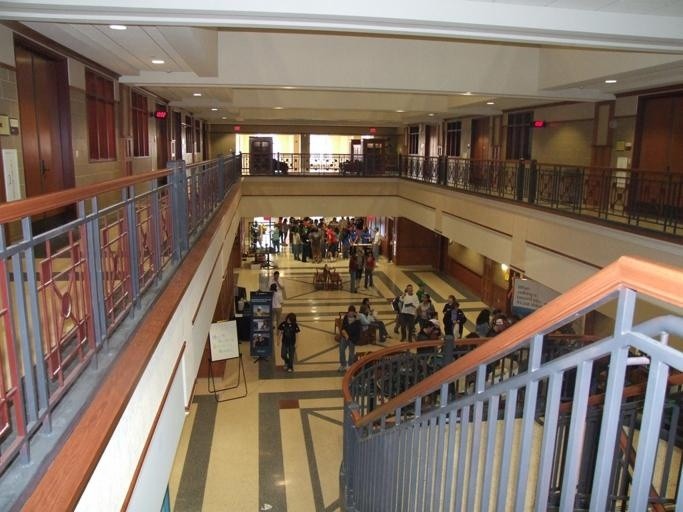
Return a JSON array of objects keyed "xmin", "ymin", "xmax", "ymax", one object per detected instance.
[
  {"xmin": 333, "ymin": 311, "xmax": 385, "ymax": 347},
  {"xmin": 312, "ymin": 264, "xmax": 343, "ymax": 291}
]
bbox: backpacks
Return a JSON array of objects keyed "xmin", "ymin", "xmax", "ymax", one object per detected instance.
[{"xmin": 391, "ymin": 297, "xmax": 398, "ymax": 312}]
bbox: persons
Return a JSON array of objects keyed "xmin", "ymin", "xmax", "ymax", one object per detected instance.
[
  {"xmin": 270, "ymin": 283, "xmax": 284, "ymax": 346},
  {"xmin": 268, "ymin": 270, "xmax": 284, "ymax": 290},
  {"xmin": 393, "ymin": 284, "xmax": 525, "ymax": 353},
  {"xmin": 277, "ymin": 312, "xmax": 301, "ymax": 373},
  {"xmin": 268, "ymin": 216, "xmax": 382, "ymax": 292},
  {"xmin": 337, "ymin": 297, "xmax": 391, "ymax": 372}
]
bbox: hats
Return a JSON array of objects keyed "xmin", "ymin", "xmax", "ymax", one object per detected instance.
[{"xmin": 348, "ymin": 305, "xmax": 355, "ymax": 311}]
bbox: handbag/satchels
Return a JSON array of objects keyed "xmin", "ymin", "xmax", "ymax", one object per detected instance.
[{"xmin": 426, "ymin": 311, "xmax": 438, "ymax": 320}]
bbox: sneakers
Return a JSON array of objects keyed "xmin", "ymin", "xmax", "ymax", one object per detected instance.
[
  {"xmin": 283, "ymin": 363, "xmax": 292, "ymax": 372},
  {"xmin": 339, "ymin": 366, "xmax": 346, "ymax": 372}
]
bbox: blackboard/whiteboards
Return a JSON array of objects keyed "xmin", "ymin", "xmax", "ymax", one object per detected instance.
[{"xmin": 210, "ymin": 320, "xmax": 239, "ymax": 362}]
